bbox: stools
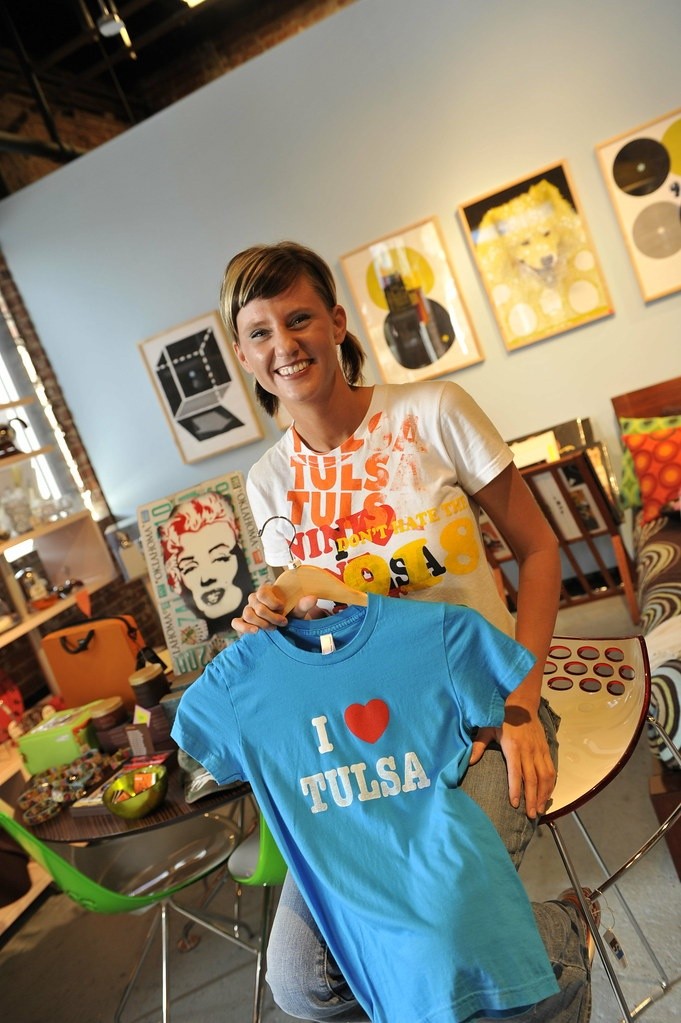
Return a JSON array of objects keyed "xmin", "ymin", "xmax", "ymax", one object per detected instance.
[{"xmin": 515, "ymin": 632, "xmax": 681, "ymax": 1023}]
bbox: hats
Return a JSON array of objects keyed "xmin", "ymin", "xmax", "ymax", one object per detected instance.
[{"xmin": 175, "ymin": 748, "xmax": 244, "ymax": 804}]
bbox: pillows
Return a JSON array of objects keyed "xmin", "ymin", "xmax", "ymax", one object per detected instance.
[
  {"xmin": 619, "ymin": 426, "xmax": 681, "ymax": 522},
  {"xmin": 615, "ymin": 415, "xmax": 681, "ymax": 513}
]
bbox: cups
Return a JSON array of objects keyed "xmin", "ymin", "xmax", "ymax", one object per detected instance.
[
  {"xmin": 89, "ymin": 696, "xmax": 129, "ymax": 733},
  {"xmin": 129, "ymin": 663, "xmax": 172, "ymax": 709}
]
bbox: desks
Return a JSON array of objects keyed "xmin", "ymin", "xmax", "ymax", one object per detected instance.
[{"xmin": 15, "ymin": 696, "xmax": 269, "ymax": 952}]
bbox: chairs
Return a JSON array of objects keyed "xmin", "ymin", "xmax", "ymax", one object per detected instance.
[{"xmin": 0, "ymin": 781, "xmax": 288, "ymax": 1023}]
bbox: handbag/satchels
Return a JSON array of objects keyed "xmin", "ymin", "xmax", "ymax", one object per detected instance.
[{"xmin": 40, "ymin": 615, "xmax": 166, "ymax": 712}]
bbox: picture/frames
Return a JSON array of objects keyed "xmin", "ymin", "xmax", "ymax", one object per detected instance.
[
  {"xmin": 457, "ymin": 160, "xmax": 615, "ymax": 351},
  {"xmin": 595, "ymin": 108, "xmax": 681, "ymax": 304},
  {"xmin": 137, "ymin": 310, "xmax": 265, "ymax": 464},
  {"xmin": 338, "ymin": 215, "xmax": 484, "ymax": 385}
]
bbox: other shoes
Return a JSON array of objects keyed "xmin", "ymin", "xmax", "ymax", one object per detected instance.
[{"xmin": 556, "ymin": 886, "xmax": 601, "ymax": 1023}]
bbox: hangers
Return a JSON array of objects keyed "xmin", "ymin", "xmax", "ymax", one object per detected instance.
[{"xmin": 257, "ymin": 514, "xmax": 367, "ymax": 621}]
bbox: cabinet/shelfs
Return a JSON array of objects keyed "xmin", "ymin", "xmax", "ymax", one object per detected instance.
[{"xmin": 0, "ymin": 398, "xmax": 119, "ymax": 717}]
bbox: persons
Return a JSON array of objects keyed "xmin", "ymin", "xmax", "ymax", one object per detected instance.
[{"xmin": 219, "ymin": 242, "xmax": 602, "ymax": 1023}]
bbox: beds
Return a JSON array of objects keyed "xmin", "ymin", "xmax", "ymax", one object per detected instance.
[{"xmin": 610, "ymin": 377, "xmax": 681, "ymax": 881}]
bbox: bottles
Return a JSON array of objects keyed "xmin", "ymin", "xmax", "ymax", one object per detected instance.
[
  {"xmin": 14, "ymin": 567, "xmax": 39, "ymax": 601},
  {"xmin": 117, "ymin": 532, "xmax": 147, "ymax": 580}
]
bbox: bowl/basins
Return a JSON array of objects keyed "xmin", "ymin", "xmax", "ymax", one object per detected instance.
[{"xmin": 102, "ymin": 765, "xmax": 168, "ymax": 819}]
